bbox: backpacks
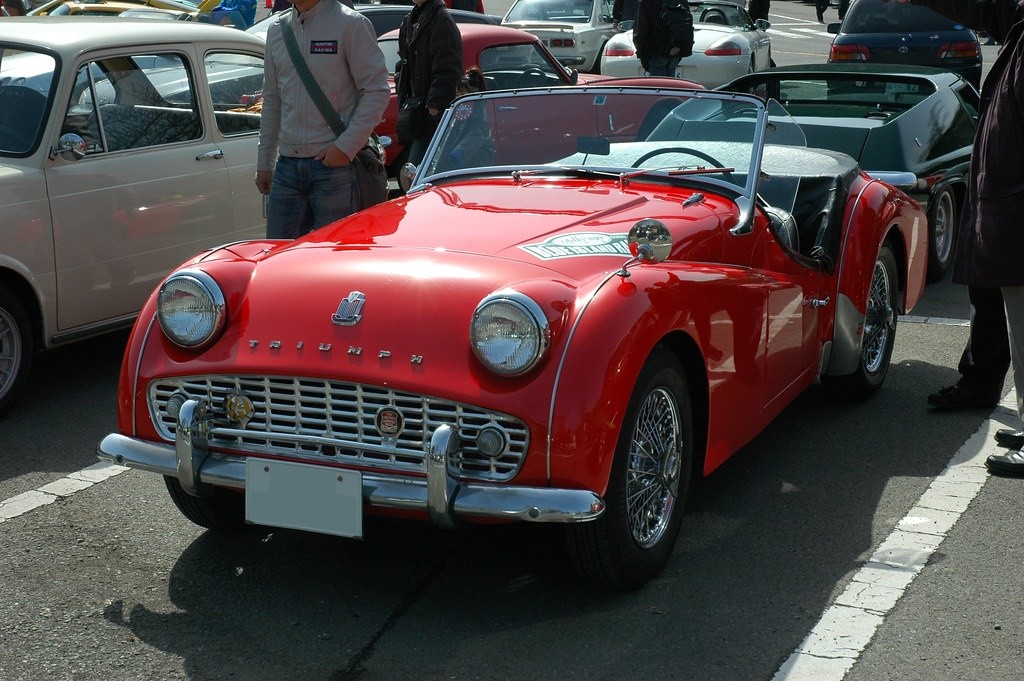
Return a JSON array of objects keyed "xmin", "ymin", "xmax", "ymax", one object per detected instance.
[{"xmin": 657, "ymin": 3, "xmax": 695, "ymax": 58}]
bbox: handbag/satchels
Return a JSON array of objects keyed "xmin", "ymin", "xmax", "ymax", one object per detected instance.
[{"xmin": 354, "ymin": 145, "xmax": 387, "ymax": 209}]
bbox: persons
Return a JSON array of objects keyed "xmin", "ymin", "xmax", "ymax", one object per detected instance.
[
  {"xmin": 198, "ymin": 0, "xmax": 258, "ymax": 30},
  {"xmin": 925, "ymin": 0, "xmax": 1024, "ymax": 479},
  {"xmin": 613, "ymin": 0, "xmax": 637, "ymax": 29},
  {"xmin": 253, "ymin": 0, "xmax": 391, "ymax": 238},
  {"xmin": 742, "ymin": 0, "xmax": 770, "ymax": 25},
  {"xmin": 815, "ymin": 0, "xmax": 830, "ymax": 24},
  {"xmin": 395, "ymin": 0, "xmax": 463, "ymax": 196},
  {"xmin": 435, "ymin": 66, "xmax": 497, "ymax": 174},
  {"xmin": 633, "ymin": 0, "xmax": 694, "ymax": 76}
]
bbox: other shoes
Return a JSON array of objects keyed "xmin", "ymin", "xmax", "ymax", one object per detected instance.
[{"xmin": 927, "ymin": 377, "xmax": 996, "ymax": 409}]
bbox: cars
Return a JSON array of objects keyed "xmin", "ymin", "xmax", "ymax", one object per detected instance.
[
  {"xmin": 823, "ymin": 0, "xmax": 983, "ymax": 107},
  {"xmin": 96, "ymin": 86, "xmax": 933, "ymax": 598},
  {"xmin": 0, "ymin": 1, "xmax": 783, "ymax": 425}
]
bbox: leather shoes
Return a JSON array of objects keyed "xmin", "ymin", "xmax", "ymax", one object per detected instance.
[
  {"xmin": 994, "ymin": 428, "xmax": 1024, "ymax": 445},
  {"xmin": 985, "ymin": 448, "xmax": 1024, "ymax": 475}
]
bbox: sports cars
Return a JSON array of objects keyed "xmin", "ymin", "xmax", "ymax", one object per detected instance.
[{"xmin": 644, "ymin": 61, "xmax": 983, "ymax": 284}]
